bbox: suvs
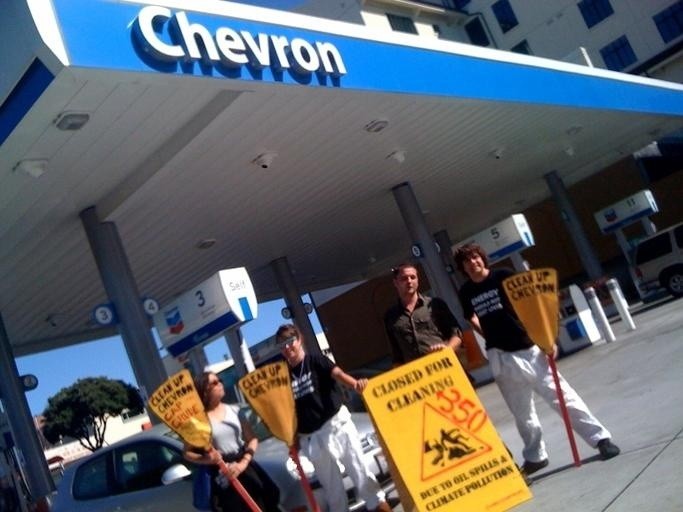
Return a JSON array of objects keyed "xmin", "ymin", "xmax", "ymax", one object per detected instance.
[{"xmin": 633, "ymin": 220, "xmax": 683, "ymax": 298}]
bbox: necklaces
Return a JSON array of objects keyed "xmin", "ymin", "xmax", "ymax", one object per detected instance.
[{"xmin": 290, "ymin": 355, "xmax": 305, "ymax": 384}]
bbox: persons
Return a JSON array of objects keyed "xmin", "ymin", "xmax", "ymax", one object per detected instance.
[
  {"xmin": 451, "ymin": 241, "xmax": 622, "ymax": 476},
  {"xmin": 276, "ymin": 323, "xmax": 393, "ymax": 512},
  {"xmin": 194, "ymin": 372, "xmax": 283, "ymax": 511},
  {"xmin": 384, "ymin": 261, "xmax": 533, "ymax": 486}
]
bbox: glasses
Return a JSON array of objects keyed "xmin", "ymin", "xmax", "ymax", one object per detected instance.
[
  {"xmin": 276, "ymin": 336, "xmax": 296, "ymax": 348},
  {"xmin": 211, "ymin": 379, "xmax": 224, "ymax": 385}
]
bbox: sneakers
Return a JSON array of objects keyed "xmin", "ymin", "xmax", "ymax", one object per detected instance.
[
  {"xmin": 596, "ymin": 438, "xmax": 620, "ymax": 461},
  {"xmin": 520, "ymin": 459, "xmax": 549, "ymax": 476}
]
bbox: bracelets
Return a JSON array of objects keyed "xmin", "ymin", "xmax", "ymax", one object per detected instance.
[{"xmin": 243, "ymin": 447, "xmax": 255, "ymax": 457}]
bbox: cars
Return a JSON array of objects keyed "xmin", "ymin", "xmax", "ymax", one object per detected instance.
[{"xmin": 48, "ymin": 403, "xmax": 395, "ymax": 512}]
